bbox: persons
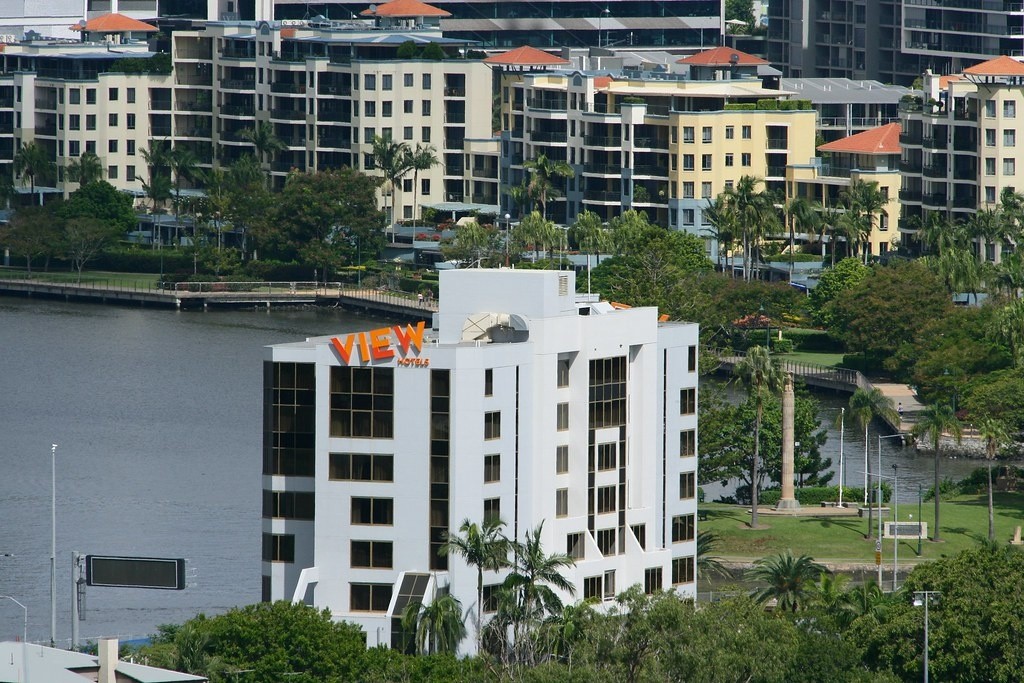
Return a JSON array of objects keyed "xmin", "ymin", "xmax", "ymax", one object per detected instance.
[
  {"xmin": 425, "ymin": 287, "xmax": 433, "ymax": 306},
  {"xmin": 897, "ymin": 402, "xmax": 904, "ymax": 418},
  {"xmin": 417, "ymin": 291, "xmax": 423, "ymax": 306}
]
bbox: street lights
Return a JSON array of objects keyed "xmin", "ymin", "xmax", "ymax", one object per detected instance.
[
  {"xmin": 912, "ymin": 589, "xmax": 944, "ymax": 683},
  {"xmin": 837, "ymin": 407, "xmax": 845, "ymax": 508},
  {"xmin": 50, "ymin": 443, "xmax": 58, "ymax": 647},
  {"xmin": 505, "ymin": 213, "xmax": 510, "ymax": 268},
  {"xmin": 878, "ymin": 433, "xmax": 913, "ymax": 596}
]
